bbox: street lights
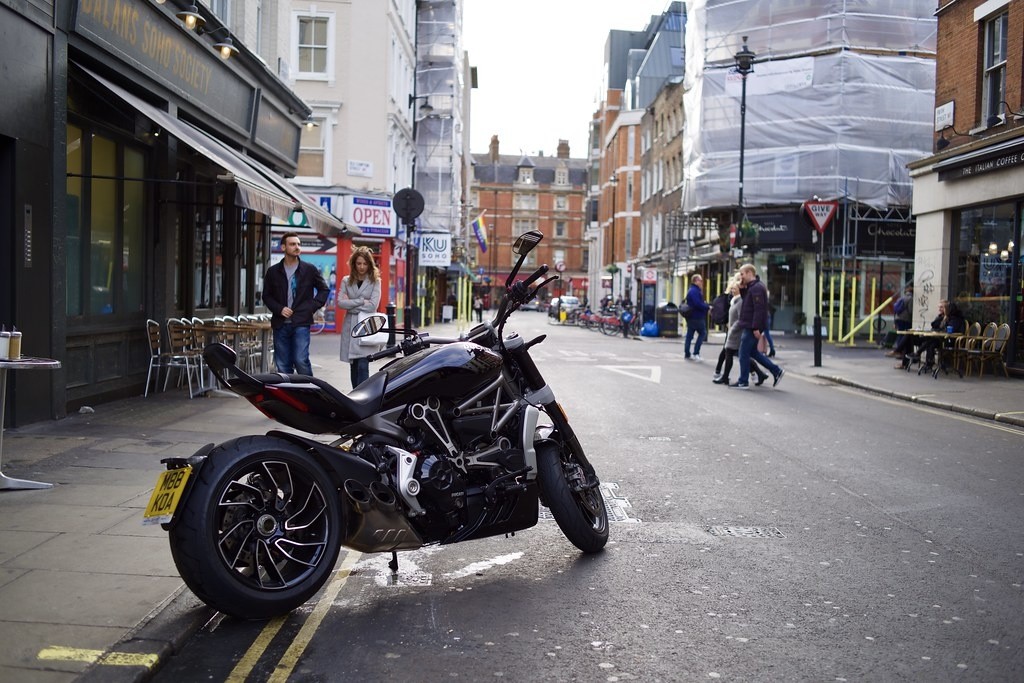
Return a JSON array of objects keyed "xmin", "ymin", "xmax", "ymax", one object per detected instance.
[
  {"xmin": 731, "ymin": 33, "xmax": 759, "ymax": 250},
  {"xmin": 404, "ymin": 93, "xmax": 434, "ymax": 342}
]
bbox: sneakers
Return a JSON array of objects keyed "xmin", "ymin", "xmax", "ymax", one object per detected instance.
[
  {"xmin": 773, "ymin": 367, "xmax": 785, "ymax": 386},
  {"xmin": 728, "ymin": 380, "xmax": 750, "ymax": 389}
]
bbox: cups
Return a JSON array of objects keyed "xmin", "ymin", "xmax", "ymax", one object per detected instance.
[{"xmin": 947, "ymin": 326, "xmax": 953, "ymax": 334}]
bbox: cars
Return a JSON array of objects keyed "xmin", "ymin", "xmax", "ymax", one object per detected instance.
[
  {"xmin": 519, "ymin": 295, "xmax": 545, "ymax": 313},
  {"xmin": 548, "ymin": 295, "xmax": 581, "ymax": 320}
]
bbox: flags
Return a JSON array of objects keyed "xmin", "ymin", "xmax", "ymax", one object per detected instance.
[{"xmin": 472, "ymin": 213, "xmax": 488, "ymax": 253}]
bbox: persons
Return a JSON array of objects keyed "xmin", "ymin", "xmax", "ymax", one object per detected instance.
[
  {"xmin": 262, "ymin": 233, "xmax": 330, "ymax": 375},
  {"xmin": 884, "ymin": 287, "xmax": 965, "ymax": 373},
  {"xmin": 712, "ymin": 262, "xmax": 784, "ymax": 387},
  {"xmin": 474, "ymin": 295, "xmax": 483, "ymax": 323},
  {"xmin": 979, "ymin": 277, "xmax": 1006, "ymax": 331},
  {"xmin": 579, "ymin": 294, "xmax": 631, "ymax": 313},
  {"xmin": 448, "ymin": 292, "xmax": 456, "ymax": 321},
  {"xmin": 685, "ymin": 274, "xmax": 713, "ymax": 361},
  {"xmin": 337, "ymin": 245, "xmax": 382, "ymax": 387}
]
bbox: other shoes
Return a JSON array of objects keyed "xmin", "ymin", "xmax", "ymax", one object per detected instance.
[
  {"xmin": 751, "ymin": 371, "xmax": 755, "ymax": 380},
  {"xmin": 686, "ymin": 355, "xmax": 694, "ymax": 360},
  {"xmin": 885, "ymin": 350, "xmax": 901, "ymax": 357},
  {"xmin": 714, "ymin": 373, "xmax": 721, "ymax": 378},
  {"xmin": 694, "ymin": 354, "xmax": 700, "ymax": 362},
  {"xmin": 894, "ymin": 362, "xmax": 908, "ymax": 369}
]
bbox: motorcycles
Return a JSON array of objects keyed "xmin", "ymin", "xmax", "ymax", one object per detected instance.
[{"xmin": 142, "ymin": 231, "xmax": 611, "ymax": 620}]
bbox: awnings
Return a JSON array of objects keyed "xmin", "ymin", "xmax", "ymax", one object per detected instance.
[
  {"xmin": 66, "ymin": 56, "xmax": 296, "ymax": 220},
  {"xmin": 179, "ymin": 117, "xmax": 362, "ymax": 241}
]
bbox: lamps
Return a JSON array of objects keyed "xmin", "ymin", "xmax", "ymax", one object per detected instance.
[
  {"xmin": 937, "ymin": 125, "xmax": 972, "ymax": 151},
  {"xmin": 289, "ymin": 108, "xmax": 319, "ymax": 128},
  {"xmin": 987, "ymin": 101, "xmax": 1024, "ymax": 128},
  {"xmin": 409, "ymin": 94, "xmax": 433, "ymax": 113},
  {"xmin": 197, "ymin": 26, "xmax": 240, "ymax": 60},
  {"xmin": 176, "ymin": 0, "xmax": 206, "ymax": 30}
]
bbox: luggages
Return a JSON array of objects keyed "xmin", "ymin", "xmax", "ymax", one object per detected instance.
[{"xmin": 757, "ymin": 333, "xmax": 767, "ymax": 356}]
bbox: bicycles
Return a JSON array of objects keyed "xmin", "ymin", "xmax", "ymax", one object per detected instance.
[{"xmin": 577, "ymin": 304, "xmax": 642, "ymax": 335}]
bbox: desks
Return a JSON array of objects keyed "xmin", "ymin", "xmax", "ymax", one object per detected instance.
[
  {"xmin": 174, "ymin": 326, "xmax": 244, "ymax": 398},
  {"xmin": 897, "ymin": 331, "xmax": 963, "ymax": 376},
  {"xmin": 0, "ymin": 356, "xmax": 61, "ymax": 490},
  {"xmin": 214, "ymin": 324, "xmax": 258, "ymax": 378},
  {"xmin": 237, "ymin": 322, "xmax": 271, "ymax": 373}
]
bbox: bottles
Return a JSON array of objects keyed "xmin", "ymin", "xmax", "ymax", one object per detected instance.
[{"xmin": 0, "ymin": 331, "xmax": 22, "ymax": 359}]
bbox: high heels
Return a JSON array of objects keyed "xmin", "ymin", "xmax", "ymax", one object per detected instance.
[
  {"xmin": 756, "ymin": 372, "xmax": 768, "ymax": 386},
  {"xmin": 767, "ymin": 351, "xmax": 775, "ymax": 358},
  {"xmin": 713, "ymin": 375, "xmax": 729, "ymax": 385}
]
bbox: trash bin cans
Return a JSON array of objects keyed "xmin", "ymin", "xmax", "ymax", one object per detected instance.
[{"xmin": 657, "ymin": 301, "xmax": 679, "ymax": 337}]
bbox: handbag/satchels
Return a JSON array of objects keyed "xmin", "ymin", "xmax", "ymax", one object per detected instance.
[{"xmin": 679, "ymin": 299, "xmax": 694, "ymax": 318}]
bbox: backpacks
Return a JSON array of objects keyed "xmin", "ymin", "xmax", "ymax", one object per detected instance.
[
  {"xmin": 893, "ymin": 294, "xmax": 913, "ymax": 314},
  {"xmin": 714, "ymin": 289, "xmax": 731, "ymax": 325}
]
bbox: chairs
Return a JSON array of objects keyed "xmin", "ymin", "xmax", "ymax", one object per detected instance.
[
  {"xmin": 145, "ymin": 313, "xmax": 273, "ymax": 399},
  {"xmin": 942, "ymin": 320, "xmax": 1011, "ymax": 380}
]
